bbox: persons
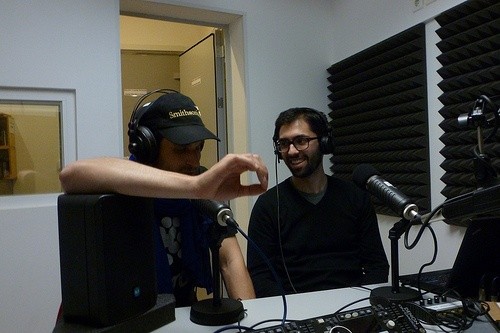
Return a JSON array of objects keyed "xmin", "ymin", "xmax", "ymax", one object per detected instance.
[
  {"xmin": 53, "ymin": 89, "xmax": 269, "ymax": 333},
  {"xmin": 247, "ymin": 107, "xmax": 390, "ymax": 299}
]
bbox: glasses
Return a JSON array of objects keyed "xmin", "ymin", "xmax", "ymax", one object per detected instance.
[{"xmin": 277, "ymin": 136, "xmax": 317, "ymax": 151}]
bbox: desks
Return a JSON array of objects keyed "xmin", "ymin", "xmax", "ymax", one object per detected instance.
[{"xmin": 148, "ymin": 282, "xmax": 500, "ymax": 333}]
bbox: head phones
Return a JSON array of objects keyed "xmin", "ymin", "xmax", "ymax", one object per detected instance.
[
  {"xmin": 272, "ymin": 108, "xmax": 336, "ymax": 163},
  {"xmin": 127, "ymin": 89, "xmax": 180, "ymax": 165}
]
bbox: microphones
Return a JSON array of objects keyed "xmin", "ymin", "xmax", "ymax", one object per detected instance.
[
  {"xmin": 191, "ymin": 197, "xmax": 240, "ymax": 228},
  {"xmin": 351, "ymin": 163, "xmax": 420, "ymax": 222}
]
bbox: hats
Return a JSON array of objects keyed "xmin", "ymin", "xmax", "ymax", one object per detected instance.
[{"xmin": 138, "ymin": 93, "xmax": 221, "ymax": 144}]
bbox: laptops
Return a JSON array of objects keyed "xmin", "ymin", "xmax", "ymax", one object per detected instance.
[{"xmin": 400, "ymin": 217, "xmax": 500, "ymax": 301}]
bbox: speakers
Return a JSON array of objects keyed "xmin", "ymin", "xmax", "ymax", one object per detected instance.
[{"xmin": 58, "ymin": 193, "xmax": 162, "ymax": 329}]
